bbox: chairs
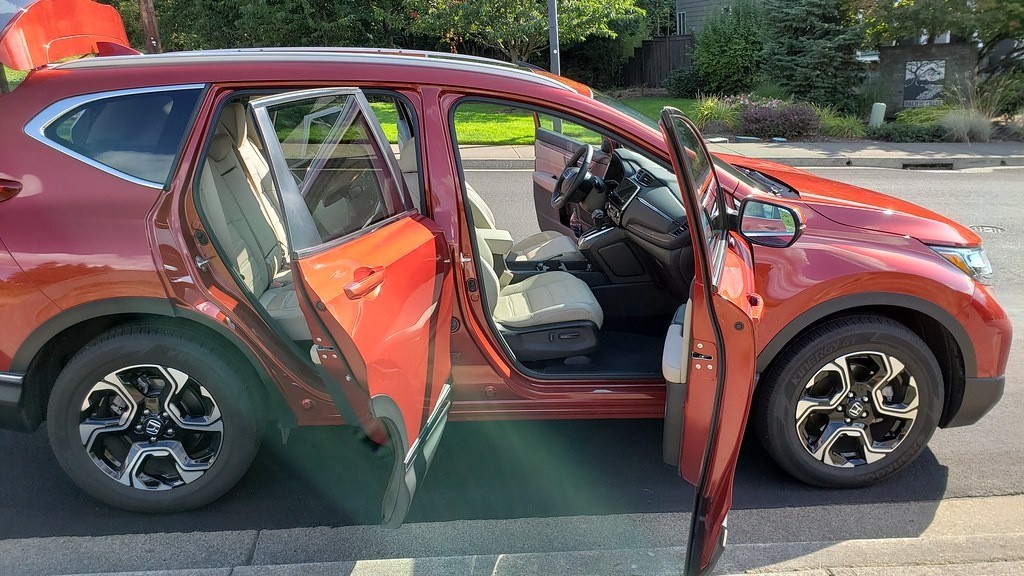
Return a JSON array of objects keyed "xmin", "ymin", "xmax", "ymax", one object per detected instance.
[{"xmin": 196, "ymin": 103, "xmax": 609, "ymax": 362}]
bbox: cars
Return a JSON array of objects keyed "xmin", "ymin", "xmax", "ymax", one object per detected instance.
[{"xmin": 1, "ymin": 1, "xmax": 1015, "ymax": 576}]
